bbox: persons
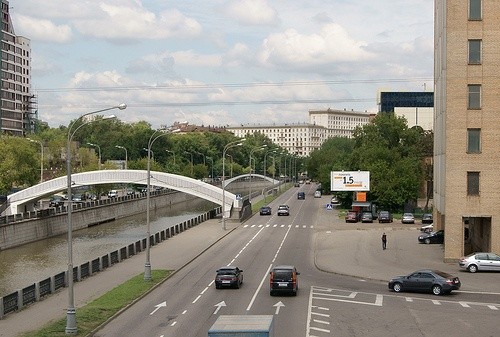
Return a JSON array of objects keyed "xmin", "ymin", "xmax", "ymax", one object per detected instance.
[{"xmin": 381, "ymin": 233, "xmax": 387, "ymax": 251}]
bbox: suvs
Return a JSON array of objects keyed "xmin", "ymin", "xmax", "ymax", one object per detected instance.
[
  {"xmin": 269, "ymin": 265, "xmax": 301, "ymax": 297},
  {"xmin": 297, "ymin": 192, "xmax": 306, "ymax": 199},
  {"xmin": 277, "ymin": 205, "xmax": 290, "ymax": 216}
]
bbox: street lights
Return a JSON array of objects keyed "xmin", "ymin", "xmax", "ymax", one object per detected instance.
[
  {"xmin": 227, "ymin": 154, "xmax": 233, "ymax": 178},
  {"xmin": 143, "ymin": 148, "xmax": 154, "ymax": 160},
  {"xmin": 165, "ymin": 149, "xmax": 175, "ymax": 164},
  {"xmin": 249, "ymin": 145, "xmax": 267, "ymax": 202},
  {"xmin": 142, "ymin": 126, "xmax": 182, "ymax": 282},
  {"xmin": 26, "ymin": 138, "xmax": 44, "ymax": 183},
  {"xmin": 115, "ymin": 145, "xmax": 128, "ymax": 170},
  {"xmin": 221, "ymin": 138, "xmax": 247, "ymax": 229},
  {"xmin": 263, "ymin": 148, "xmax": 292, "ymax": 202},
  {"xmin": 87, "ymin": 143, "xmax": 101, "ymax": 170},
  {"xmin": 63, "ymin": 104, "xmax": 129, "ymax": 334}
]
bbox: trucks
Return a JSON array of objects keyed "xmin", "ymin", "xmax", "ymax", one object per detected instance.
[{"xmin": 208, "ymin": 315, "xmax": 273, "ymax": 337}]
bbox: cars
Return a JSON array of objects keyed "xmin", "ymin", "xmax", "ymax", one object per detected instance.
[
  {"xmin": 389, "ymin": 269, "xmax": 461, "ymax": 296},
  {"xmin": 294, "ymin": 178, "xmax": 313, "ymax": 188},
  {"xmin": 314, "ymin": 190, "xmax": 322, "ymax": 198},
  {"xmin": 213, "ymin": 267, "xmax": 244, "ymax": 289},
  {"xmin": 418, "ymin": 229, "xmax": 443, "ymax": 245},
  {"xmin": 420, "ymin": 222, "xmax": 433, "ymax": 233},
  {"xmin": 343, "ymin": 209, "xmax": 393, "ymax": 223},
  {"xmin": 458, "ymin": 252, "xmax": 500, "ymax": 272},
  {"xmin": 260, "ymin": 206, "xmax": 272, "ymax": 215},
  {"xmin": 422, "ymin": 214, "xmax": 433, "ymax": 223},
  {"xmin": 49, "ymin": 185, "xmax": 165, "ymax": 207},
  {"xmin": 402, "ymin": 213, "xmax": 415, "ymax": 224}
]
